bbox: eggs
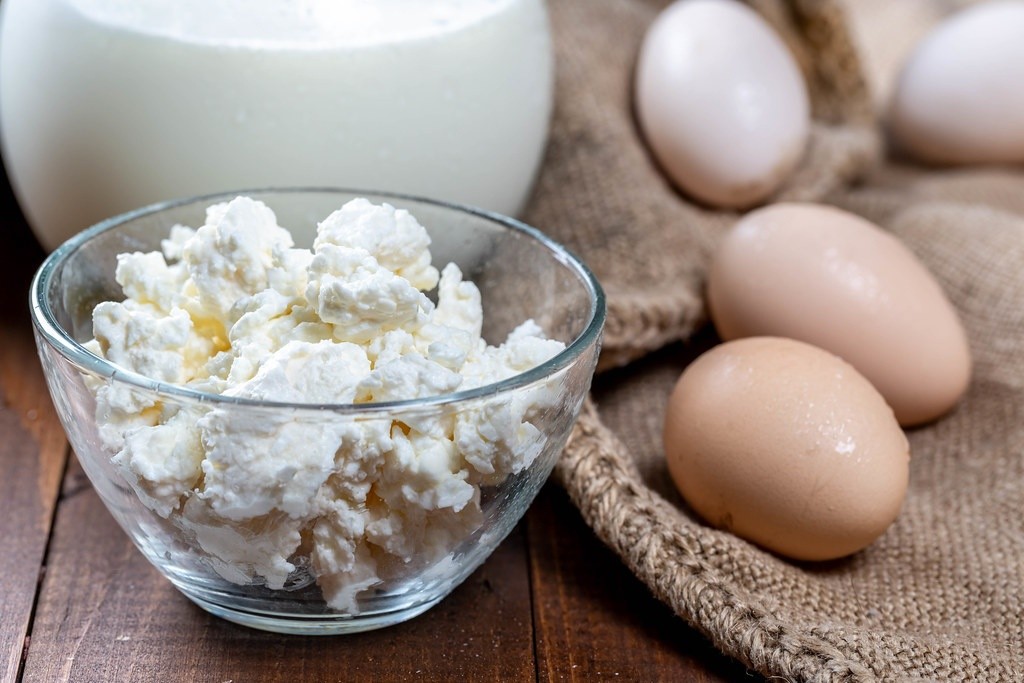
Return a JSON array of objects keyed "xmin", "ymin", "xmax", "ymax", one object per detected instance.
[
  {"xmin": 896, "ymin": 3, "xmax": 1024, "ymax": 162},
  {"xmin": 633, "ymin": 0, "xmax": 811, "ymax": 209},
  {"xmin": 663, "ymin": 201, "xmax": 971, "ymax": 561}
]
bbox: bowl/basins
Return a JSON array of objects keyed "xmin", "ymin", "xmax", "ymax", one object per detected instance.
[
  {"xmin": 29, "ymin": 186, "xmax": 608, "ymax": 635},
  {"xmin": 0, "ymin": 2, "xmax": 557, "ymax": 305}
]
